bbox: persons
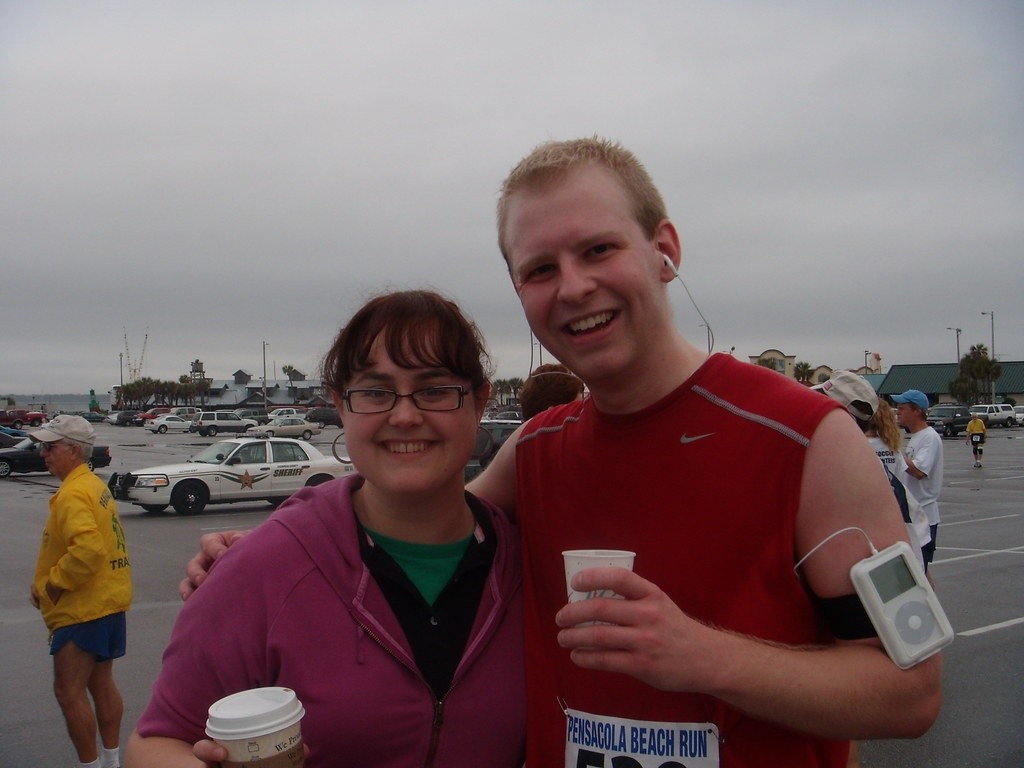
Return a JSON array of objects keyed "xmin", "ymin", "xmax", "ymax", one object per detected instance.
[
  {"xmin": 889, "ymin": 389, "xmax": 944, "ymax": 591},
  {"xmin": 519, "ymin": 364, "xmax": 587, "ymax": 424},
  {"xmin": 179, "ymin": 133, "xmax": 942, "ymax": 768},
  {"xmin": 885, "ymin": 465, "xmax": 934, "ymax": 577},
  {"xmin": 965, "ymin": 412, "xmax": 987, "ymax": 470},
  {"xmin": 863, "ymin": 397, "xmax": 909, "ymax": 482},
  {"xmin": 807, "ymin": 370, "xmax": 878, "ymax": 433},
  {"xmin": 122, "ymin": 283, "xmax": 526, "ymax": 768},
  {"xmin": 29, "ymin": 414, "xmax": 132, "ymax": 768}
]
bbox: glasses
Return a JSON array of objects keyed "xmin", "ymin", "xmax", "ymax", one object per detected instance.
[
  {"xmin": 41, "ymin": 441, "xmax": 74, "ymax": 452},
  {"xmin": 342, "ymin": 385, "xmax": 471, "ymax": 414}
]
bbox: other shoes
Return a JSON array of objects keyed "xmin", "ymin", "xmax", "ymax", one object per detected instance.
[
  {"xmin": 974, "ymin": 462, "xmax": 978, "ymax": 467},
  {"xmin": 977, "ymin": 462, "xmax": 982, "ymax": 468}
]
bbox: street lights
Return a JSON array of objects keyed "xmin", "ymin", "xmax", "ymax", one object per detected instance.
[
  {"xmin": 262, "ymin": 340, "xmax": 270, "ymax": 403},
  {"xmin": 981, "ymin": 311, "xmax": 996, "ymax": 404},
  {"xmin": 945, "ymin": 327, "xmax": 962, "ymax": 363}
]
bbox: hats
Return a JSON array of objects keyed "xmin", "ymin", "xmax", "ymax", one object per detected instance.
[
  {"xmin": 890, "ymin": 390, "xmax": 929, "ymax": 410},
  {"xmin": 809, "ymin": 371, "xmax": 879, "ymax": 421},
  {"xmin": 29, "ymin": 415, "xmax": 96, "ymax": 445}
]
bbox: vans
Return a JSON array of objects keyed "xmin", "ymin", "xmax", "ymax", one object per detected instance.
[
  {"xmin": 969, "ymin": 404, "xmax": 1016, "ymax": 428},
  {"xmin": 1013, "ymin": 406, "xmax": 1024, "ymax": 426}
]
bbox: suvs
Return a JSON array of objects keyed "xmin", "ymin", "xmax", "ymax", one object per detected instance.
[{"xmin": 190, "ymin": 410, "xmax": 258, "ymax": 438}]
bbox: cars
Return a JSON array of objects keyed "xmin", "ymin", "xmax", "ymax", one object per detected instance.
[
  {"xmin": 246, "ymin": 418, "xmax": 322, "ymax": 441},
  {"xmin": 0, "ymin": 409, "xmax": 47, "ymax": 430},
  {"xmin": 0, "ymin": 425, "xmax": 29, "ymax": 449},
  {"xmin": 0, "ymin": 436, "xmax": 112, "ymax": 478},
  {"xmin": 927, "ymin": 402, "xmax": 970, "ymax": 437},
  {"xmin": 77, "ymin": 407, "xmax": 344, "ymax": 429},
  {"xmin": 462, "ymin": 405, "xmax": 523, "ymax": 484},
  {"xmin": 144, "ymin": 416, "xmax": 193, "ymax": 434},
  {"xmin": 112, "ymin": 431, "xmax": 360, "ymax": 516}
]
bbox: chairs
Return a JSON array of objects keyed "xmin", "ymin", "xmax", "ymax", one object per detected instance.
[{"xmin": 240, "ymin": 450, "xmax": 252, "ymax": 464}]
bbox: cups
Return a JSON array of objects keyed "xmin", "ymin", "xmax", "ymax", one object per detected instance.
[
  {"xmin": 562, "ymin": 550, "xmax": 636, "ymax": 650},
  {"xmin": 205, "ymin": 687, "xmax": 305, "ymax": 768}
]
